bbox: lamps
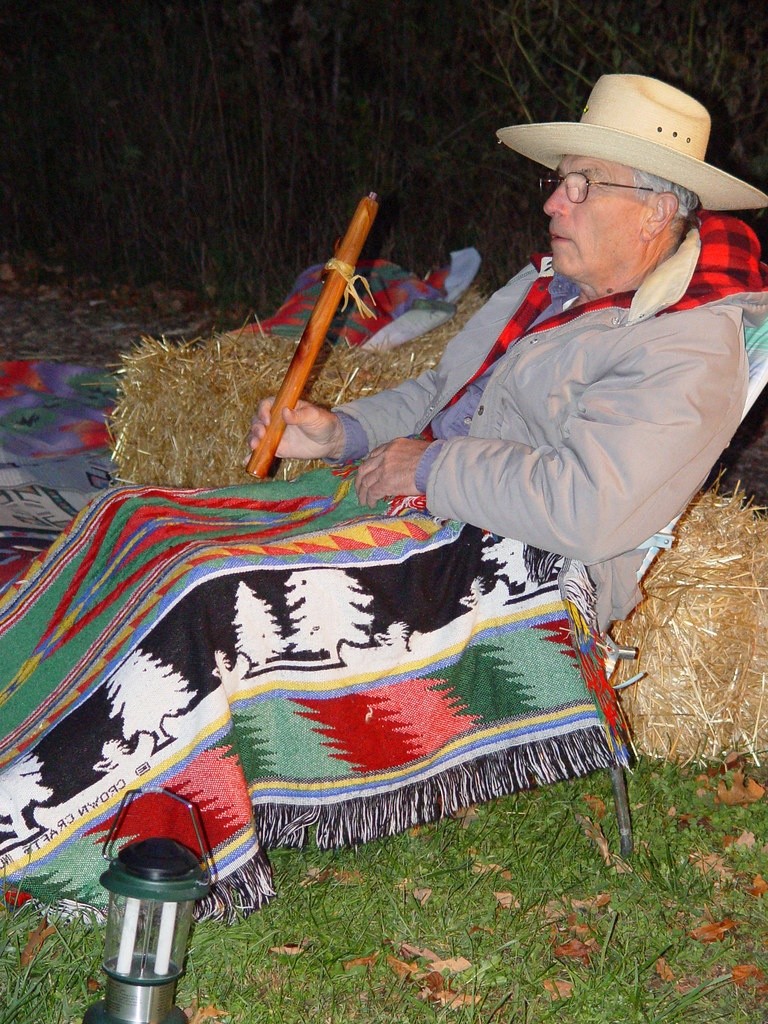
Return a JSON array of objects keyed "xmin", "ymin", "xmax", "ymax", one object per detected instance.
[{"xmin": 78, "ymin": 783, "xmax": 225, "ymax": 1024}]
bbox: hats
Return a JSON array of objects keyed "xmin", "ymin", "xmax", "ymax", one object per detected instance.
[{"xmin": 495, "ymin": 73, "xmax": 768, "ymax": 211}]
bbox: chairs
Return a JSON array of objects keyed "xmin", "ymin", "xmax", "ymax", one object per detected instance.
[{"xmin": 587, "ymin": 505, "xmax": 689, "ymax": 856}]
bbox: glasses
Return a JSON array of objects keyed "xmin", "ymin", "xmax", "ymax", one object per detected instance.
[{"xmin": 538, "ymin": 169, "xmax": 653, "ymax": 204}]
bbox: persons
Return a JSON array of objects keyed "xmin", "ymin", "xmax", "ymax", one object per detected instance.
[{"xmin": 0, "ymin": 73, "xmax": 754, "ymax": 872}]
